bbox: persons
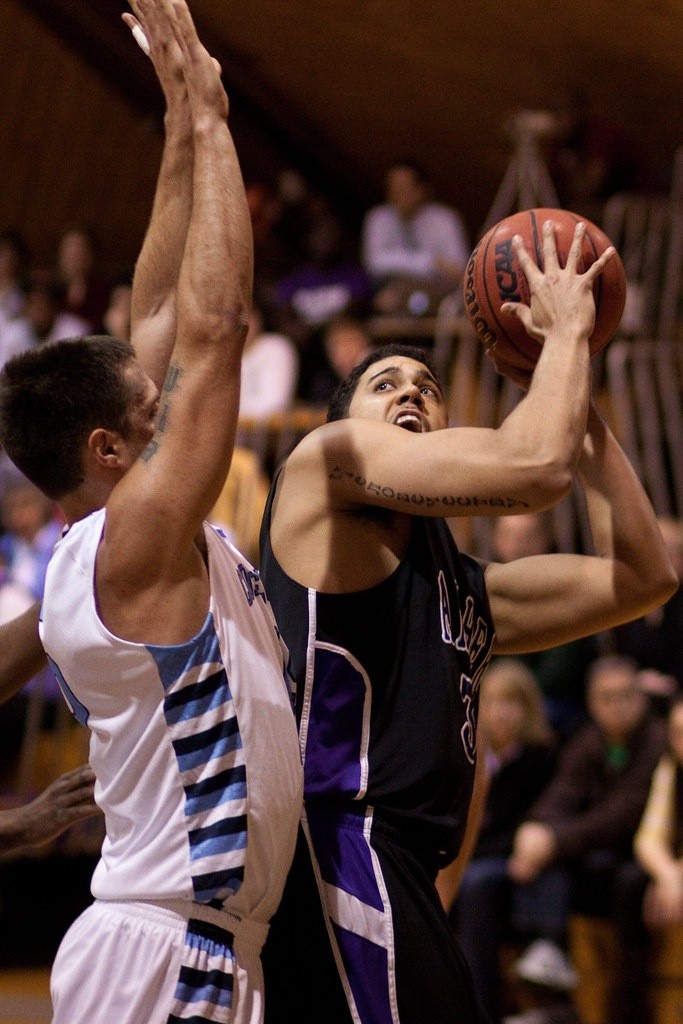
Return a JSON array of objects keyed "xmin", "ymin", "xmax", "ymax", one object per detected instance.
[
  {"xmin": 2, "ymin": 0, "xmax": 305, "ymax": 1024},
  {"xmin": 633, "ymin": 698, "xmax": 683, "ymax": 982},
  {"xmin": 442, "ymin": 660, "xmax": 561, "ymax": 1024},
  {"xmin": 0, "ymin": 166, "xmax": 680, "ymax": 695},
  {"xmin": 258, "ymin": 217, "xmax": 681, "ymax": 1024},
  {"xmin": 507, "ymin": 656, "xmax": 663, "ymax": 997},
  {"xmin": 0, "ymin": 598, "xmax": 103, "ymax": 863}
]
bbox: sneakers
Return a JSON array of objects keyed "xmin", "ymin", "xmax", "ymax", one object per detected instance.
[{"xmin": 511, "ymin": 937, "xmax": 583, "ymax": 989}]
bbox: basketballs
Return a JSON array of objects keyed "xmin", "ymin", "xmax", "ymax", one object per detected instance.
[{"xmin": 462, "ymin": 210, "xmax": 626, "ymax": 371}]
